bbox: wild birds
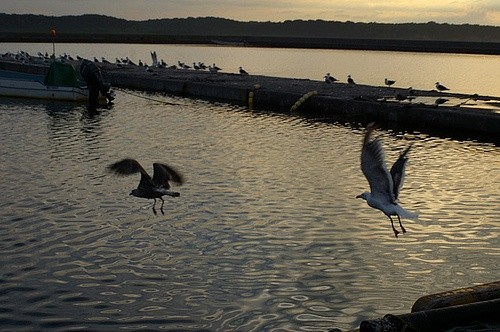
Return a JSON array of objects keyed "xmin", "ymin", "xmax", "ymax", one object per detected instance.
[
  {"xmin": 435, "ymin": 82, "xmax": 449, "ymax": 93},
  {"xmin": 432, "ymin": 98, "xmax": 449, "ymax": 106},
  {"xmin": 356, "ymin": 131, "xmax": 419, "ymax": 237},
  {"xmin": 348, "ymin": 75, "xmax": 356, "ymax": 85},
  {"xmin": 0, "ymin": 50, "xmax": 222, "ymax": 74},
  {"xmin": 238, "ymin": 65, "xmax": 246, "ymax": 74},
  {"xmin": 323, "ymin": 72, "xmax": 339, "ymax": 85},
  {"xmin": 107, "ymin": 158, "xmax": 183, "ymax": 217},
  {"xmin": 384, "ymin": 78, "xmax": 395, "ymax": 86}
]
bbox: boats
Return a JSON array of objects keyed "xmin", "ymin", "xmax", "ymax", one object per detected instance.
[{"xmin": 0, "ymin": 61, "xmax": 114, "ymax": 103}]
